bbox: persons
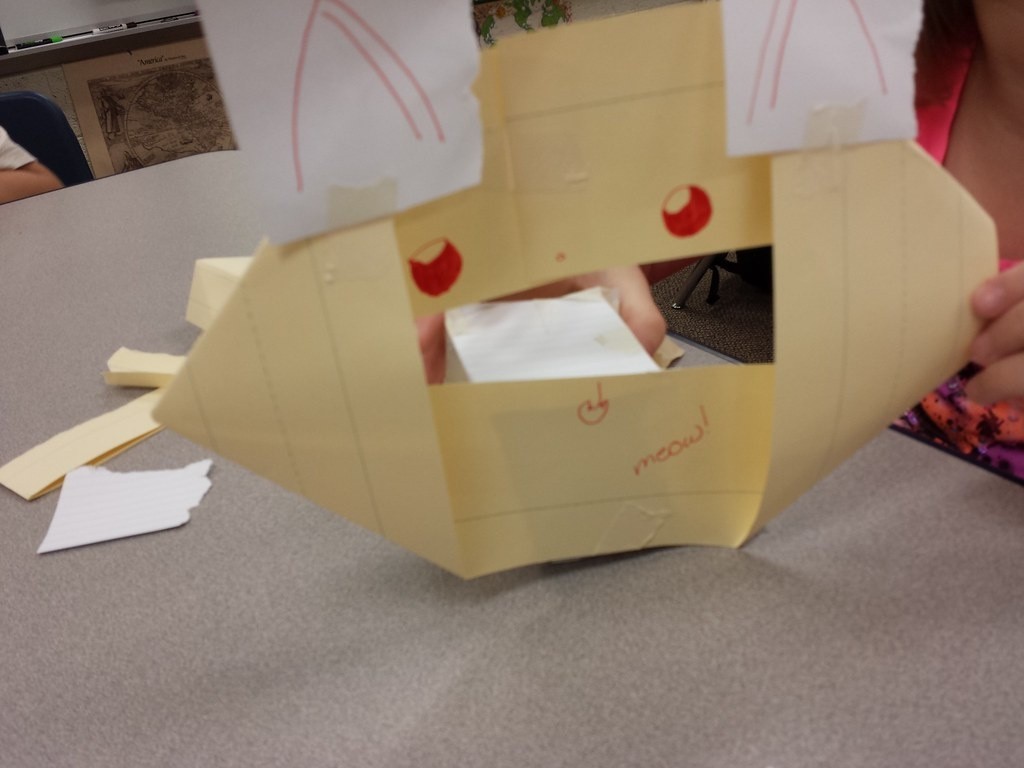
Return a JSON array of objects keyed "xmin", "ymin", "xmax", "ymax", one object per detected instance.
[
  {"xmin": 415, "ymin": 0, "xmax": 1024, "ymax": 489},
  {"xmin": 1, "ymin": 127, "xmax": 64, "ymax": 206}
]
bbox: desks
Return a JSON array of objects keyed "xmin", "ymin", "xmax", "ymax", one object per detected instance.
[{"xmin": 0, "ymin": 150, "xmax": 1024, "ymax": 767}]
